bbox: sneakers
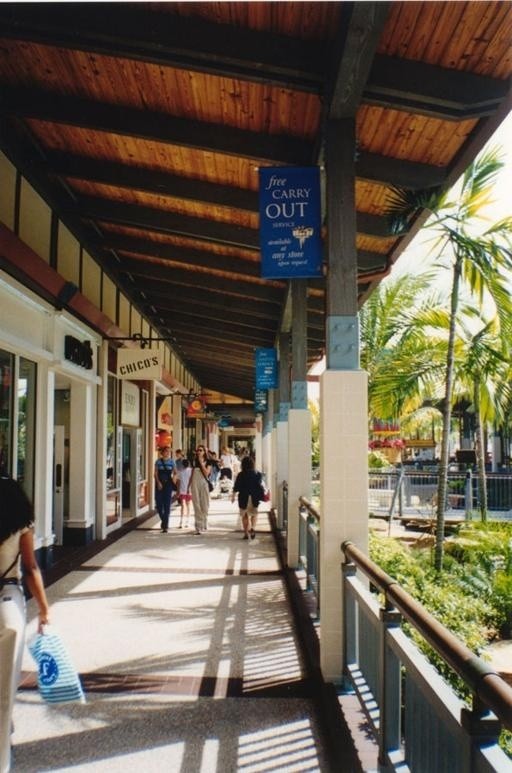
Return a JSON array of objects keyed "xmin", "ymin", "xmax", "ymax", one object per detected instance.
[
  {"xmin": 162, "ymin": 525, "xmax": 207, "ymax": 535},
  {"xmin": 243, "ymin": 528, "xmax": 258, "ymax": 540}
]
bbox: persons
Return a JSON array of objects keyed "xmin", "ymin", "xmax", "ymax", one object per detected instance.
[
  {"xmin": 153, "ymin": 439, "xmax": 263, "ymax": 540},
  {"xmin": 0, "ymin": 466, "xmax": 52, "ymax": 773}
]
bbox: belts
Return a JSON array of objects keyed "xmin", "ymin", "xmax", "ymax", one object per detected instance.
[{"xmin": 0, "ymin": 576, "xmax": 22, "ymax": 587}]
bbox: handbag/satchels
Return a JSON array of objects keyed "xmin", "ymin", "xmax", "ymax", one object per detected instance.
[
  {"xmin": 172, "ymin": 480, "xmax": 177, "ymax": 491},
  {"xmin": 262, "ymin": 490, "xmax": 270, "ymax": 501},
  {"xmin": 208, "ymin": 480, "xmax": 214, "ymax": 492}
]
